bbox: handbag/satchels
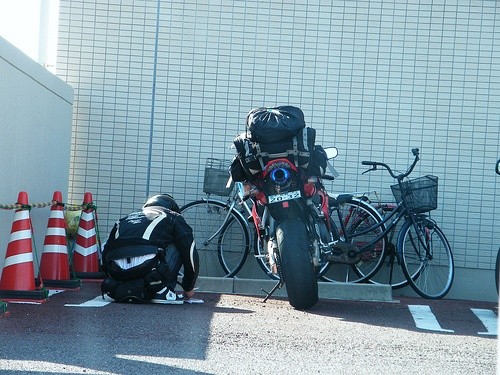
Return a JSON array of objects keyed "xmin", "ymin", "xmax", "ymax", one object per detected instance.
[
  {"xmin": 244, "ymin": 105, "xmax": 306, "ymax": 144},
  {"xmin": 233, "ymin": 126, "xmax": 316, "ymax": 176}
]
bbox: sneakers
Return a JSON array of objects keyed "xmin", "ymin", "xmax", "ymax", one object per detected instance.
[{"xmin": 151, "ymin": 285, "xmax": 183, "ymax": 304}]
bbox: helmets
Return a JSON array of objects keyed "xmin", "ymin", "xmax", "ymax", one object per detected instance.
[{"xmin": 143, "ymin": 194, "xmax": 181, "ymax": 214}]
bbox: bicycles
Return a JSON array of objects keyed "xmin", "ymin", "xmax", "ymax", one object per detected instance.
[{"xmin": 179, "ymin": 149, "xmax": 455, "ymax": 300}]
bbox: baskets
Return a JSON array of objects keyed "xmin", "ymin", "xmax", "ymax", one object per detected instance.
[
  {"xmin": 204, "ymin": 158, "xmax": 234, "ymax": 195},
  {"xmin": 390, "ymin": 174, "xmax": 438, "ymax": 214}
]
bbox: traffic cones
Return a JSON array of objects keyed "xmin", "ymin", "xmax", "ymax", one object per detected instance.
[
  {"xmin": 0, "ymin": 190, "xmax": 49, "ymax": 300},
  {"xmin": 71, "ymin": 191, "xmax": 105, "ymax": 279},
  {"xmin": 35, "ymin": 190, "xmax": 82, "ymax": 288}
]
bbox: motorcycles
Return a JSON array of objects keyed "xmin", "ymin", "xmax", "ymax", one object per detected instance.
[{"xmin": 238, "ymin": 146, "xmax": 339, "ymax": 310}]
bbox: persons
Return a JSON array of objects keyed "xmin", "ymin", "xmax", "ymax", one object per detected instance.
[{"xmin": 101, "ymin": 193, "xmax": 200, "ymax": 304}]
